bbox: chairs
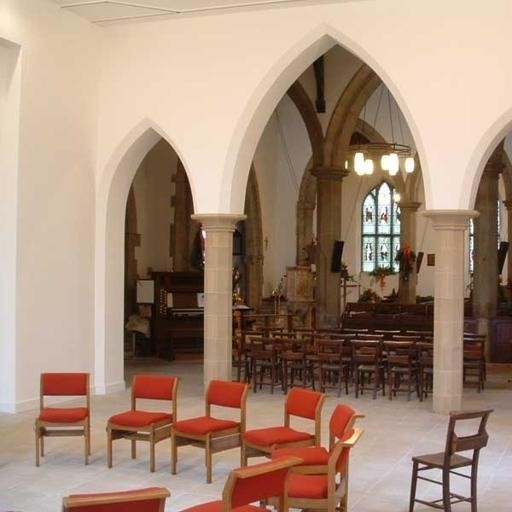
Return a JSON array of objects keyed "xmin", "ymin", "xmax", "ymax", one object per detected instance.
[
  {"xmin": 408, "ymin": 408, "xmax": 494, "ymax": 512},
  {"xmin": 272, "ymin": 403, "xmax": 366, "ymax": 476},
  {"xmin": 237, "ymin": 301, "xmax": 488, "ymax": 402},
  {"xmin": 258, "ymin": 428, "xmax": 365, "ymax": 512},
  {"xmin": 34, "ymin": 372, "xmax": 92, "ymax": 466},
  {"xmin": 240, "ymin": 386, "xmax": 327, "ymax": 471},
  {"xmin": 62, "ymin": 486, "xmax": 171, "ymax": 512},
  {"xmin": 179, "ymin": 455, "xmax": 303, "ymax": 512},
  {"xmin": 170, "ymin": 380, "xmax": 250, "ymax": 485},
  {"xmin": 106, "ymin": 374, "xmax": 179, "ymax": 472}
]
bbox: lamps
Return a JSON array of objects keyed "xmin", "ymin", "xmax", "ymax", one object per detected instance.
[{"xmin": 342, "ymin": 82, "xmax": 416, "ymax": 177}]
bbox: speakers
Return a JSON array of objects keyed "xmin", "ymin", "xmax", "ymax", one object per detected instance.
[
  {"xmin": 498, "ymin": 241, "xmax": 510, "ymax": 274},
  {"xmin": 416, "ymin": 252, "xmax": 424, "ymax": 272},
  {"xmin": 330, "ymin": 240, "xmax": 345, "ymax": 273}
]
